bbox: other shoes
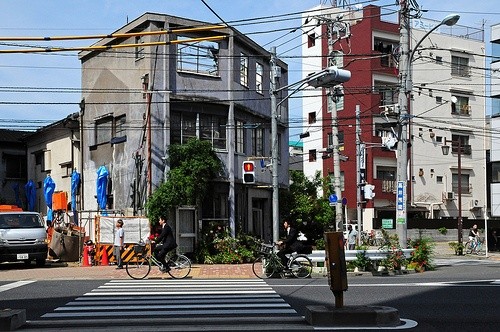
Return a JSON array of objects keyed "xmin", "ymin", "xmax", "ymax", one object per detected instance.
[
  {"xmin": 160, "ymin": 266, "xmax": 171, "ymax": 273},
  {"xmin": 116, "ymin": 265, "xmax": 123, "ymax": 269},
  {"xmin": 282, "ymin": 267, "xmax": 290, "ymax": 272}
]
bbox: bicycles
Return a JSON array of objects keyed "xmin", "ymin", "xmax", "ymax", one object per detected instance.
[
  {"xmin": 465, "ymin": 234, "xmax": 485, "ymax": 256},
  {"xmin": 342, "ymin": 219, "xmax": 386, "ymax": 246},
  {"xmin": 126, "ymin": 239, "xmax": 192, "ymax": 280},
  {"xmin": 253, "ymin": 242, "xmax": 314, "ymax": 279}
]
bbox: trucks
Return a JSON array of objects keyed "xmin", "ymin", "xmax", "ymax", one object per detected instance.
[{"xmin": 0, "ymin": 211, "xmax": 51, "ymax": 267}]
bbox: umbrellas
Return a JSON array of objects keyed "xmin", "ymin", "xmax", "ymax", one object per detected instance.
[
  {"xmin": 71, "ymin": 169, "xmax": 81, "ymax": 212},
  {"xmin": 95, "ymin": 165, "xmax": 111, "ymax": 218},
  {"xmin": 23, "ymin": 179, "xmax": 37, "ymax": 213},
  {"xmin": 12, "ymin": 181, "xmax": 23, "ymax": 210},
  {"xmin": 43, "ymin": 174, "xmax": 57, "ymax": 226}
]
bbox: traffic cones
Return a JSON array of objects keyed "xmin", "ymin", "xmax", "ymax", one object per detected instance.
[
  {"xmin": 80, "ymin": 246, "xmax": 92, "ymax": 267},
  {"xmin": 100, "ymin": 246, "xmax": 110, "ymax": 266}
]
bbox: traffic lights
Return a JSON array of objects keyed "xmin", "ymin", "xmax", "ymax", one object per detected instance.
[{"xmin": 242, "ymin": 161, "xmax": 256, "ymax": 185}]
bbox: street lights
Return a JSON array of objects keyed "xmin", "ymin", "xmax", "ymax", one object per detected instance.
[
  {"xmin": 396, "ymin": 13, "xmax": 461, "ymax": 251},
  {"xmin": 269, "ymin": 46, "xmax": 351, "ymax": 249}
]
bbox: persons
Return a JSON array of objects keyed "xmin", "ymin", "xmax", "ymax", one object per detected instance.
[
  {"xmin": 345, "ymin": 224, "xmax": 359, "ymax": 249},
  {"xmin": 275, "ymin": 220, "xmax": 299, "ymax": 273},
  {"xmin": 150, "ymin": 217, "xmax": 178, "ymax": 274},
  {"xmin": 467, "ymin": 223, "xmax": 480, "ymax": 255},
  {"xmin": 115, "ymin": 220, "xmax": 125, "ymax": 268}
]
